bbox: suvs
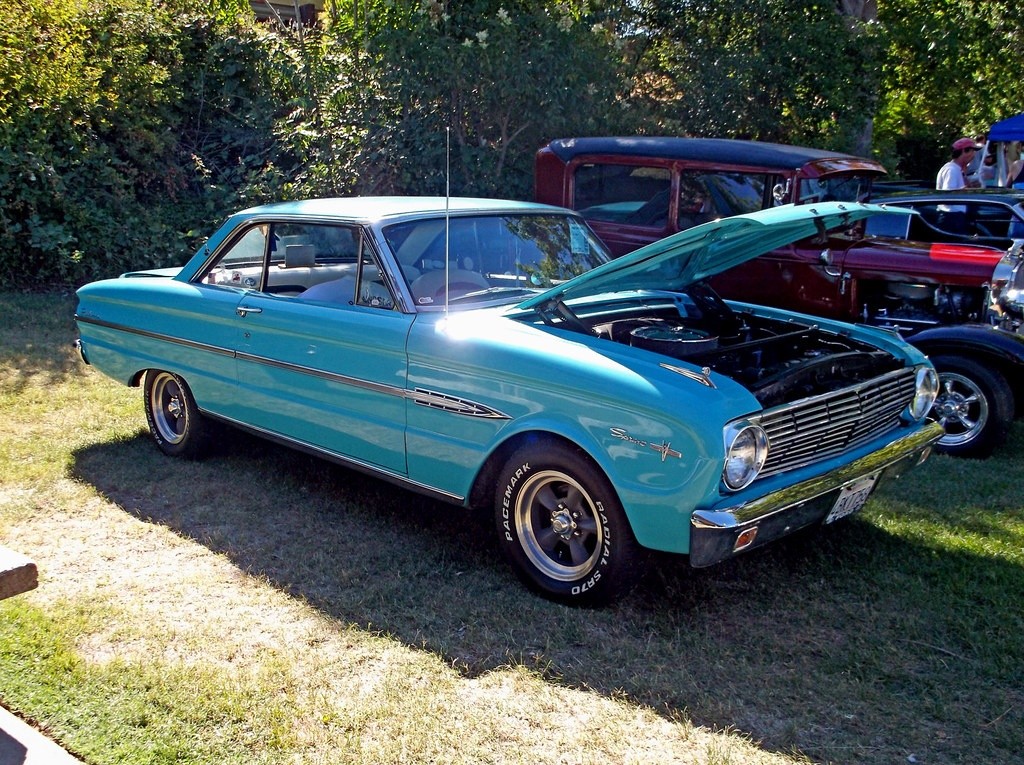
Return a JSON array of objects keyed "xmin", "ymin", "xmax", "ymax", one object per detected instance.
[{"xmin": 534, "ymin": 132, "xmax": 1024, "ymax": 458}]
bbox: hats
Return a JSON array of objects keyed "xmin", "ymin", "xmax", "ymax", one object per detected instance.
[{"xmin": 952, "ymin": 138, "xmax": 983, "ymax": 151}]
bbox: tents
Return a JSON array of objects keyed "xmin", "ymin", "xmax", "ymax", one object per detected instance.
[{"xmin": 980, "ymin": 114, "xmax": 1024, "ymax": 174}]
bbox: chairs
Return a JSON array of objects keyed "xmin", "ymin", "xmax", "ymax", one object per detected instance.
[
  {"xmin": 297, "ymin": 275, "xmax": 393, "ymax": 305},
  {"xmin": 410, "ymin": 269, "xmax": 489, "ymax": 304}
]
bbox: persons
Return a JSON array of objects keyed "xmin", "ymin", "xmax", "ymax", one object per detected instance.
[{"xmin": 934, "ymin": 135, "xmax": 1024, "ymax": 190}]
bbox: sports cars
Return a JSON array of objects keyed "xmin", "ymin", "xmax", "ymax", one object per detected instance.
[{"xmin": 71, "ymin": 193, "xmax": 947, "ymax": 611}]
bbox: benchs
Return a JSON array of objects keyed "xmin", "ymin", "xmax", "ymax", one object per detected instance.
[{"xmin": 218, "ymin": 264, "xmax": 421, "ymax": 296}]
